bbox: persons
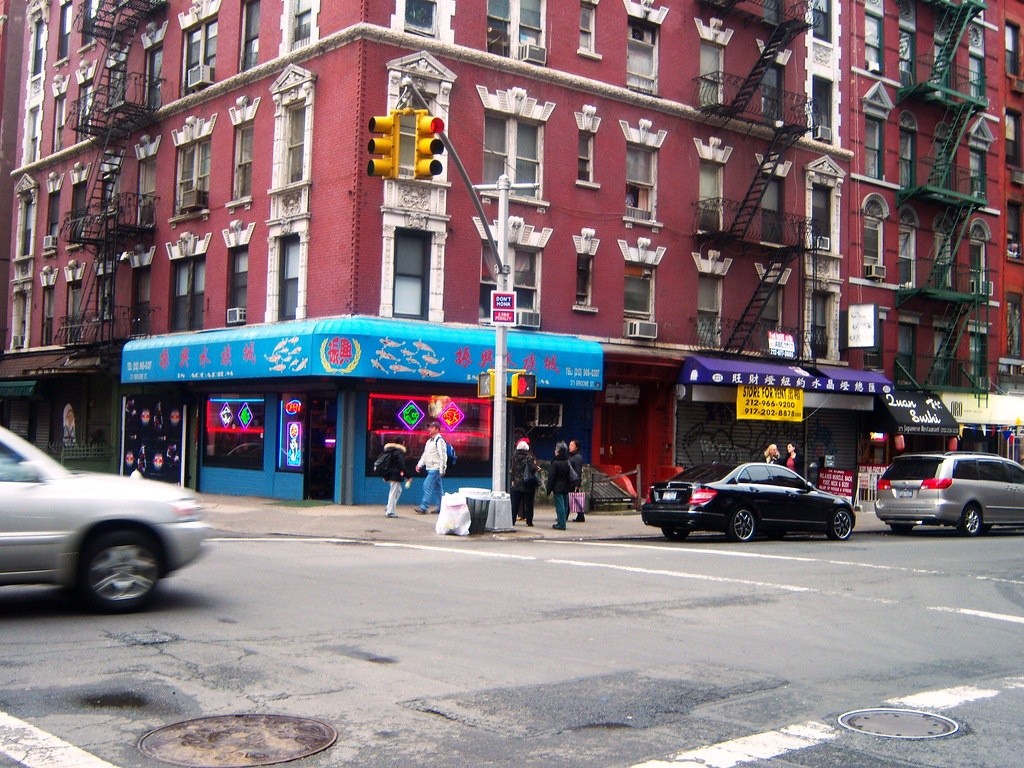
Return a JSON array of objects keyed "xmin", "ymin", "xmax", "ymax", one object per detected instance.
[
  {"xmin": 414, "ymin": 422, "xmax": 448, "ymax": 514},
  {"xmin": 382, "ymin": 436, "xmax": 413, "ymax": 518},
  {"xmin": 509, "ymin": 437, "xmax": 536, "ymax": 526},
  {"xmin": 545, "ymin": 440, "xmax": 585, "ymax": 529},
  {"xmin": 757, "ymin": 440, "xmax": 805, "ymax": 478}
]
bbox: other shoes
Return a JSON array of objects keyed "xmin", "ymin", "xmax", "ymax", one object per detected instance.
[
  {"xmin": 573, "ymin": 513, "xmax": 585, "ymax": 522},
  {"xmin": 414, "ymin": 507, "xmax": 426, "ymax": 515},
  {"xmin": 385, "ymin": 513, "xmax": 398, "ymax": 518},
  {"xmin": 431, "ymin": 508, "xmax": 440, "ymax": 513},
  {"xmin": 552, "ymin": 522, "xmax": 566, "ymax": 530}
]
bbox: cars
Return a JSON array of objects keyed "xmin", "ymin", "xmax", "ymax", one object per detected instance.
[
  {"xmin": 226, "ymin": 442, "xmax": 264, "ymax": 459},
  {"xmin": 641, "ymin": 462, "xmax": 855, "ymax": 543},
  {"xmin": 0, "ymin": 425, "xmax": 207, "ymax": 612}
]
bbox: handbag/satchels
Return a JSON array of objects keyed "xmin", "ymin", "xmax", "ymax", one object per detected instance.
[
  {"xmin": 569, "ymin": 487, "xmax": 586, "ymax": 513},
  {"xmin": 568, "ymin": 461, "xmax": 582, "ymax": 488},
  {"xmin": 524, "ymin": 457, "xmax": 542, "ymax": 488}
]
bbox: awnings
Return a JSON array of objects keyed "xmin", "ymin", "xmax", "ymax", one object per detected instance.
[
  {"xmin": 680, "ymin": 355, "xmax": 895, "ymax": 395},
  {"xmin": 880, "ymin": 392, "xmax": 960, "ymax": 436},
  {"xmin": 940, "ymin": 391, "xmax": 1024, "ymax": 425}
]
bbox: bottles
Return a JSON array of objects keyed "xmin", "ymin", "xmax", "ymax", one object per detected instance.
[{"xmin": 405, "ymin": 481, "xmax": 411, "ymax": 489}]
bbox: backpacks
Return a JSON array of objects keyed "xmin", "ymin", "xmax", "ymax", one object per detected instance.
[
  {"xmin": 373, "ymin": 449, "xmax": 399, "ymax": 476},
  {"xmin": 436, "ymin": 437, "xmax": 458, "ymax": 466}
]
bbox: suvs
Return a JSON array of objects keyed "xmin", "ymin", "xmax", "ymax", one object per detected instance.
[{"xmin": 874, "ymin": 451, "xmax": 1024, "ymax": 537}]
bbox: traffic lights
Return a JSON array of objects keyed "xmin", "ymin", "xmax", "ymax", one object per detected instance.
[
  {"xmin": 413, "ymin": 110, "xmax": 444, "ymax": 180},
  {"xmin": 367, "ymin": 110, "xmax": 400, "ymax": 181},
  {"xmin": 511, "ymin": 373, "xmax": 537, "ymax": 399},
  {"xmin": 478, "ymin": 371, "xmax": 495, "ymax": 398}
]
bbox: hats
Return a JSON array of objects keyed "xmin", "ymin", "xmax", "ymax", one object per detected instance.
[
  {"xmin": 427, "ymin": 422, "xmax": 440, "ymax": 430},
  {"xmin": 517, "ymin": 438, "xmax": 530, "ymax": 450}
]
